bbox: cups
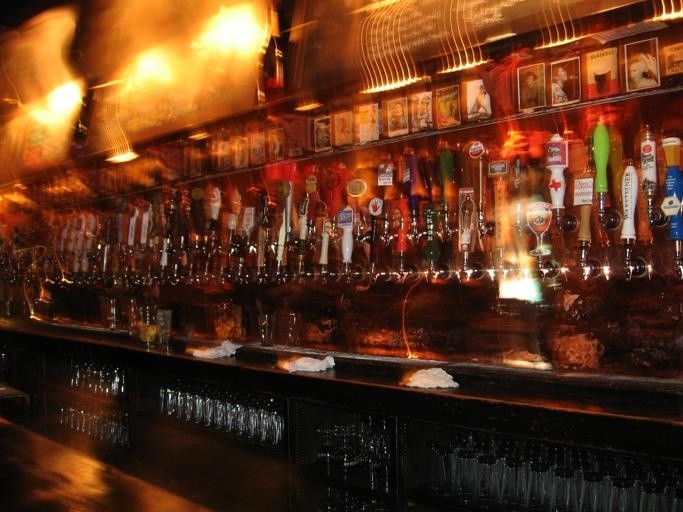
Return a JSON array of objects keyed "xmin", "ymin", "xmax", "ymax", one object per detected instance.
[
  {"xmin": 158, "ymin": 388, "xmax": 285, "ymax": 448},
  {"xmin": 432, "ymin": 433, "xmax": 683, "ymax": 512},
  {"xmin": 258, "ymin": 313, "xmax": 275, "ymax": 345},
  {"xmin": 288, "ymin": 313, "xmax": 305, "ymax": 344},
  {"xmin": 594, "ymin": 70, "xmax": 612, "ymax": 94}
]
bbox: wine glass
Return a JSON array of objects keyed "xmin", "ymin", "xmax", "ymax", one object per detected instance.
[
  {"xmin": 60, "ymin": 406, "xmax": 129, "ymax": 446},
  {"xmin": 68, "ymin": 358, "xmax": 127, "ymax": 400},
  {"xmin": 134, "ymin": 304, "xmax": 158, "ymax": 351}
]
